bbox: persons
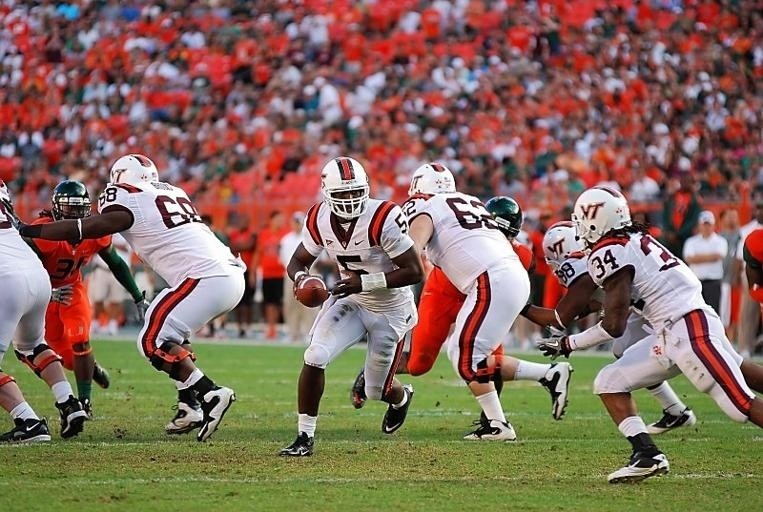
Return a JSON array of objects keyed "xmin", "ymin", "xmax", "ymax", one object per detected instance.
[
  {"xmin": 1, "ymin": 153, "xmax": 246, "ymax": 442},
  {"xmin": 278, "ymin": 157, "xmax": 423, "ymax": 456},
  {"xmin": 351, "ymin": 197, "xmax": 532, "ymax": 427},
  {"xmin": 535, "ymin": 186, "xmax": 763, "ymax": 483},
  {"xmin": 403, "ymin": 163, "xmax": 572, "ymax": 442},
  {"xmin": 0, "ymin": 178, "xmax": 88, "ymax": 444},
  {"xmin": 679, "ymin": 208, "xmax": 728, "ymax": 318},
  {"xmin": 31, "ymin": 181, "xmax": 150, "ymax": 421},
  {"xmin": 519, "ymin": 221, "xmax": 763, "ymax": 434},
  {"xmin": 0, "ymin": 1, "xmax": 762, "ymax": 189}
]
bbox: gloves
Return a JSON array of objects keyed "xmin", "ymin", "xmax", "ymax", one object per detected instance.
[
  {"xmin": 49, "ymin": 282, "xmax": 75, "ymax": 307},
  {"xmin": 134, "ymin": 290, "xmax": 152, "ymax": 320},
  {"xmin": 534, "ymin": 334, "xmax": 572, "ymax": 362}
]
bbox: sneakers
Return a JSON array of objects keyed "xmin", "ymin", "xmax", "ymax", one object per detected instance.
[
  {"xmin": 192, "ymin": 385, "xmax": 237, "ymax": 443},
  {"xmin": 0, "ymin": 417, "xmax": 52, "ymax": 443},
  {"xmin": 279, "ymin": 432, "xmax": 315, "ymax": 457},
  {"xmin": 381, "ymin": 383, "xmax": 416, "ymax": 434},
  {"xmin": 92, "ymin": 360, "xmax": 110, "ymax": 389},
  {"xmin": 54, "ymin": 394, "xmax": 88, "ymax": 439},
  {"xmin": 349, "ymin": 368, "xmax": 368, "ymax": 410},
  {"xmin": 605, "ymin": 450, "xmax": 671, "ymax": 484},
  {"xmin": 165, "ymin": 401, "xmax": 205, "ymax": 435},
  {"xmin": 78, "ymin": 396, "xmax": 93, "ymax": 417},
  {"xmin": 646, "ymin": 407, "xmax": 698, "ymax": 434},
  {"xmin": 463, "ymin": 418, "xmax": 517, "ymax": 441},
  {"xmin": 538, "ymin": 361, "xmax": 575, "ymax": 421}
]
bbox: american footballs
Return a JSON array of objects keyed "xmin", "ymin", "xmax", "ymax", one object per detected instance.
[{"xmin": 295, "ymin": 275, "xmax": 330, "ymax": 308}]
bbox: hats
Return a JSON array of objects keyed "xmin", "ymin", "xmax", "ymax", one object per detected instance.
[{"xmin": 698, "ymin": 209, "xmax": 715, "ymax": 225}]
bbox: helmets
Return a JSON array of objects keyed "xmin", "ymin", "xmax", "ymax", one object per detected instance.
[
  {"xmin": 109, "ymin": 153, "xmax": 159, "ymax": 186},
  {"xmin": 542, "ymin": 219, "xmax": 589, "ymax": 270},
  {"xmin": 571, "ymin": 183, "xmax": 633, "ymax": 251},
  {"xmin": 318, "ymin": 155, "xmax": 371, "ymax": 220},
  {"xmin": 50, "ymin": 180, "xmax": 92, "ymax": 221},
  {"xmin": 407, "ymin": 162, "xmax": 456, "ymax": 198},
  {"xmin": 485, "ymin": 194, "xmax": 523, "ymax": 236}
]
